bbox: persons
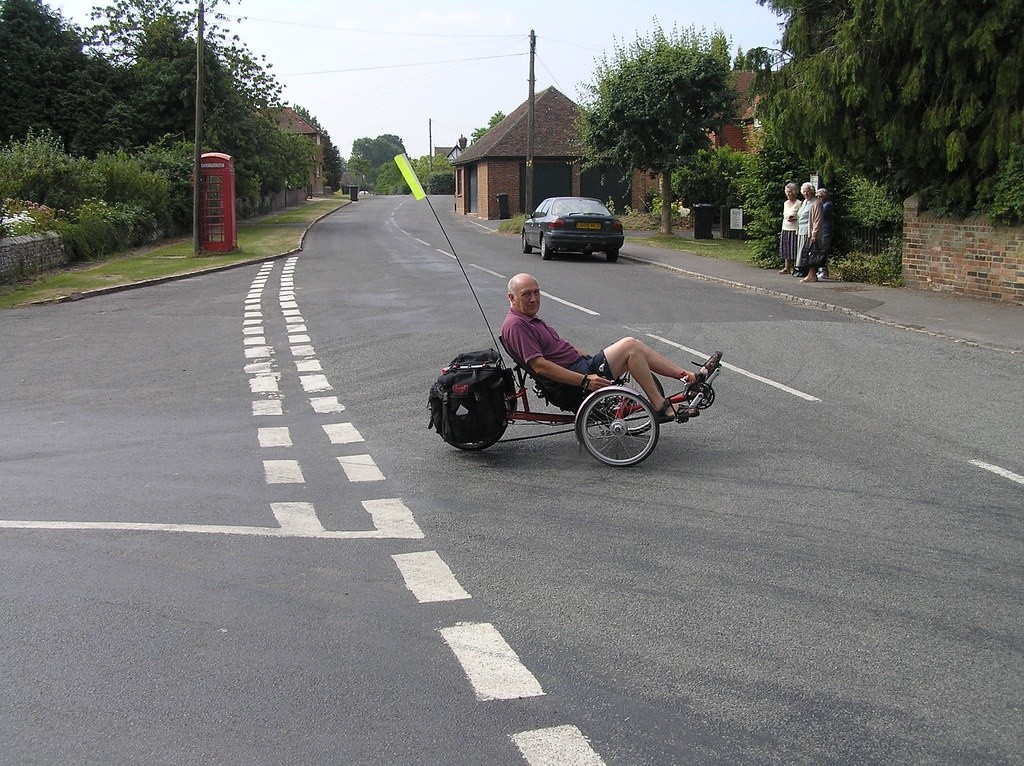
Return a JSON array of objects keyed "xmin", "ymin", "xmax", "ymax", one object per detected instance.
[
  {"xmin": 500, "ymin": 273, "xmax": 724, "ymax": 420},
  {"xmin": 812, "ymin": 188, "xmax": 834, "ymax": 279},
  {"xmin": 787, "ymin": 182, "xmax": 824, "ymax": 283},
  {"xmin": 779, "ymin": 183, "xmax": 803, "ymax": 275}
]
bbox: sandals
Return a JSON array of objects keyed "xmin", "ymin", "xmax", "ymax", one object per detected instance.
[
  {"xmin": 654, "ymin": 398, "xmax": 701, "ymax": 425},
  {"xmin": 686, "ymin": 351, "xmax": 723, "ymax": 389}
]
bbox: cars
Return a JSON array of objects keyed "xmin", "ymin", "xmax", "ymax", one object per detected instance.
[{"xmin": 522, "ymin": 197, "xmax": 625, "ymax": 262}]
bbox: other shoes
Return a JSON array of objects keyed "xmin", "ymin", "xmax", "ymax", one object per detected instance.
[{"xmin": 778, "ymin": 267, "xmax": 830, "ymax": 282}]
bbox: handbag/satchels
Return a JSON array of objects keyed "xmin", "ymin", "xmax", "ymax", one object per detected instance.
[
  {"xmin": 426, "ymin": 348, "xmax": 517, "ymax": 446},
  {"xmin": 801, "ymin": 241, "xmax": 827, "ymax": 269}
]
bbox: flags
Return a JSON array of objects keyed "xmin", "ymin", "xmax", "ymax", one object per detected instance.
[{"xmin": 394, "ymin": 153, "xmax": 426, "ymax": 201}]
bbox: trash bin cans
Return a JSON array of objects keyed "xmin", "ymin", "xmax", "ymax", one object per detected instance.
[
  {"xmin": 496, "ymin": 193, "xmax": 511, "ymax": 220},
  {"xmin": 349, "ymin": 185, "xmax": 358, "ymax": 201},
  {"xmin": 341, "ymin": 186, "xmax": 349, "ymax": 194},
  {"xmin": 692, "ymin": 203, "xmax": 716, "ymax": 239}
]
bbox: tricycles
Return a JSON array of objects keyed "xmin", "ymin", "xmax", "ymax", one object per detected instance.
[{"xmin": 395, "ymin": 152, "xmax": 723, "ymax": 467}]
